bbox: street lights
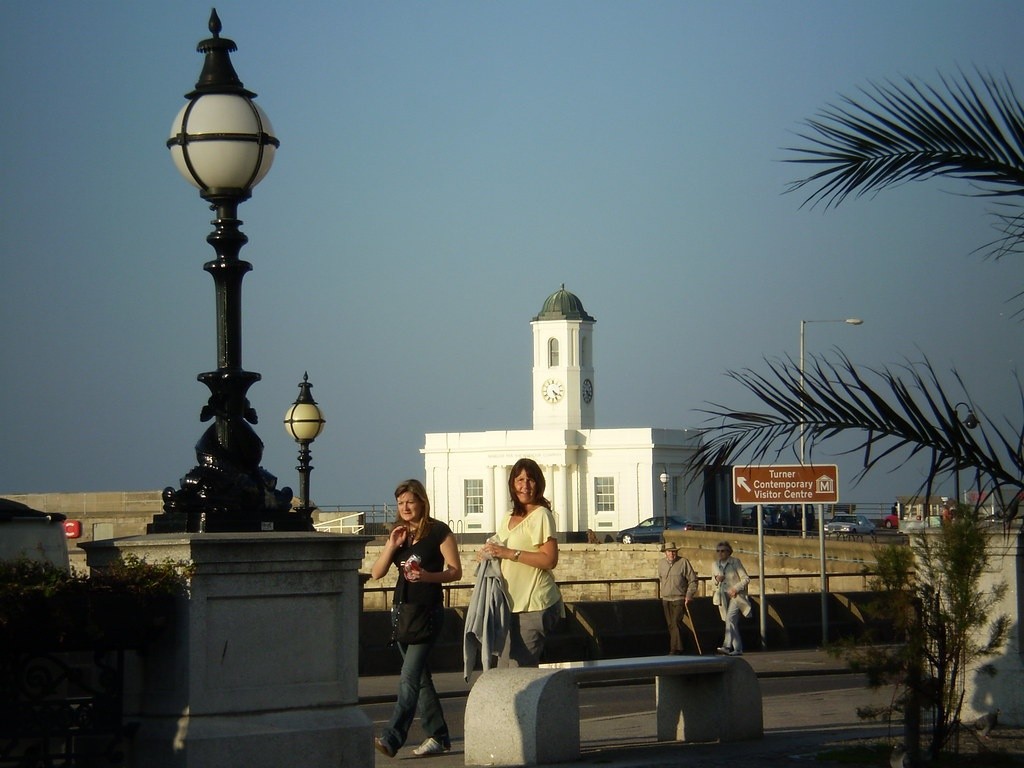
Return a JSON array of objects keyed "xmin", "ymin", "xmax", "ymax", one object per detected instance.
[
  {"xmin": 282, "ymin": 370, "xmax": 327, "ymax": 532},
  {"xmin": 659, "ymin": 466, "xmax": 669, "ymax": 529},
  {"xmin": 147, "ymin": 6, "xmax": 316, "ymax": 533},
  {"xmin": 800, "ymin": 317, "xmax": 865, "ymax": 539},
  {"xmin": 951, "ymin": 402, "xmax": 981, "ymax": 505}
]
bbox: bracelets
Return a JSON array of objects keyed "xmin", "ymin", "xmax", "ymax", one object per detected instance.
[{"xmin": 512, "ymin": 550, "xmax": 521, "ymax": 562}]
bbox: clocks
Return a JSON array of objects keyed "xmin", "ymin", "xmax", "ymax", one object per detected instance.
[
  {"xmin": 542, "ymin": 376, "xmax": 565, "ymax": 403},
  {"xmin": 583, "ymin": 379, "xmax": 593, "ymax": 404}
]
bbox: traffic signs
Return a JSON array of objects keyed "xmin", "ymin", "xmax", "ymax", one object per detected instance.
[{"xmin": 731, "ymin": 462, "xmax": 840, "ymax": 504}]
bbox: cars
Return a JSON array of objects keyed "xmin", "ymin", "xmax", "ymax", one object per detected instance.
[
  {"xmin": 824, "ymin": 514, "xmax": 876, "ymax": 536},
  {"xmin": 616, "ymin": 515, "xmax": 705, "ymax": 545},
  {"xmin": 882, "ymin": 514, "xmax": 898, "ymax": 529}
]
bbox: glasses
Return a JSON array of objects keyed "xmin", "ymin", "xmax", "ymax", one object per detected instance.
[{"xmin": 716, "ymin": 550, "xmax": 730, "ymax": 553}]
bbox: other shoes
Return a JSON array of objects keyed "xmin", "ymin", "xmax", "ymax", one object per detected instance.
[
  {"xmin": 729, "ymin": 650, "xmax": 743, "ymax": 656},
  {"xmin": 717, "ymin": 647, "xmax": 730, "ymax": 655},
  {"xmin": 669, "ymin": 649, "xmax": 681, "ymax": 655}
]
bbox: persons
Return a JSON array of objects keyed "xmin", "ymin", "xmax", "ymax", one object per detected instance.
[
  {"xmin": 712, "ymin": 542, "xmax": 750, "ymax": 656},
  {"xmin": 371, "ymin": 479, "xmax": 462, "ymax": 758},
  {"xmin": 658, "ymin": 542, "xmax": 696, "ymax": 655},
  {"xmin": 942, "ymin": 505, "xmax": 951, "ymax": 525},
  {"xmin": 476, "ymin": 459, "xmax": 563, "ymax": 671}
]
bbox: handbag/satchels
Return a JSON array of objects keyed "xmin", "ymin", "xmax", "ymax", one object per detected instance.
[{"xmin": 713, "ymin": 584, "xmax": 722, "ymax": 606}]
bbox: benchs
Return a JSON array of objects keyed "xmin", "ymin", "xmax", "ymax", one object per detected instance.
[{"xmin": 463, "ymin": 655, "xmax": 764, "ymax": 766}]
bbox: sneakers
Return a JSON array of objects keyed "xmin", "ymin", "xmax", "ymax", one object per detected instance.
[
  {"xmin": 412, "ymin": 737, "xmax": 449, "ymax": 755},
  {"xmin": 374, "ymin": 737, "xmax": 396, "ymax": 758}
]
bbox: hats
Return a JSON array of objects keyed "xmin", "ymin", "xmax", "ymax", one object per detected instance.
[{"xmin": 660, "ymin": 542, "xmax": 680, "ymax": 552}]
